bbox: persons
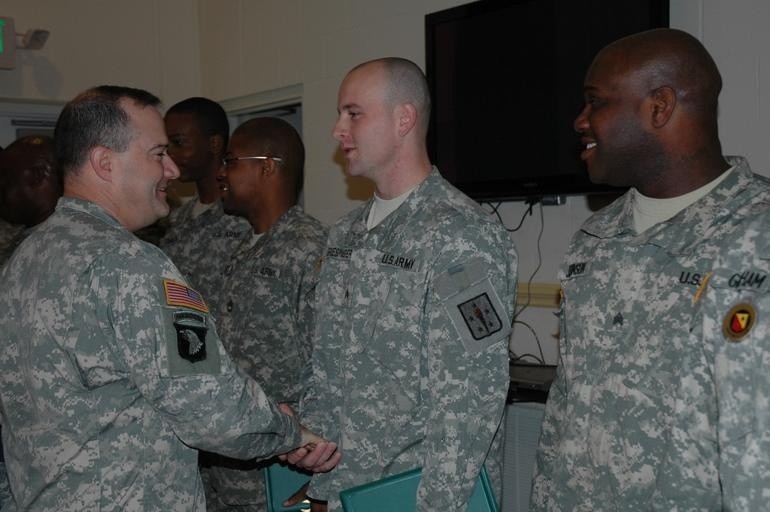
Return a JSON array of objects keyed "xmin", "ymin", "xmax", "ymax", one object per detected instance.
[
  {"xmin": 273, "ymin": 54, "xmax": 521, "ymax": 512},
  {"xmin": 198, "ymin": 115, "xmax": 332, "ymax": 512},
  {"xmin": 528, "ymin": 24, "xmax": 770, "ymax": 512},
  {"xmin": 1, "ymin": 136, "xmax": 62, "ymax": 260},
  {"xmin": 0, "ymin": 85, "xmax": 331, "ymax": 512},
  {"xmin": 149, "ymin": 94, "xmax": 252, "ymax": 292}
]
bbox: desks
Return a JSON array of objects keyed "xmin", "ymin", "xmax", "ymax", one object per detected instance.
[{"xmin": 499, "ymin": 396, "xmax": 546, "ymax": 511}]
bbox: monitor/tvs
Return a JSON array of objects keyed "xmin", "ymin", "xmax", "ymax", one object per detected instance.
[{"xmin": 424, "ymin": 0, "xmax": 671, "ymax": 203}]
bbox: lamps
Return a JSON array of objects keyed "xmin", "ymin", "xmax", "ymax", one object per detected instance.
[{"xmin": 0, "ymin": 12, "xmax": 49, "ymax": 69}]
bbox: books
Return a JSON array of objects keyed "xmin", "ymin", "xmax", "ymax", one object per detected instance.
[
  {"xmin": 338, "ymin": 460, "xmax": 499, "ymax": 512},
  {"xmin": 263, "ymin": 451, "xmax": 313, "ymax": 512}
]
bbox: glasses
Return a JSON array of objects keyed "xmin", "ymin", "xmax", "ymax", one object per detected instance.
[{"xmin": 222, "ymin": 154, "xmax": 283, "ymax": 167}]
